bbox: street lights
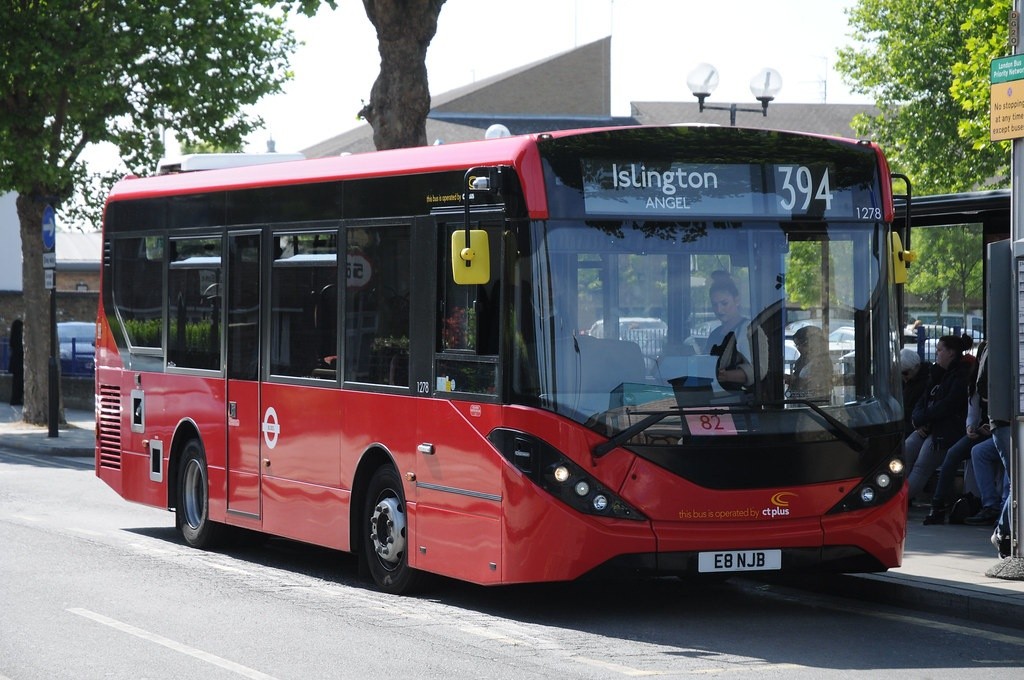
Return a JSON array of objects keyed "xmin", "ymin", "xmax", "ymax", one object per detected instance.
[{"xmin": 687, "ymin": 64, "xmax": 784, "ymax": 128}]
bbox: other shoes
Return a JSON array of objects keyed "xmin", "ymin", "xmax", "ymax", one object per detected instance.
[
  {"xmin": 923, "ymin": 504, "xmax": 953, "ymax": 525},
  {"xmin": 963, "ymin": 506, "xmax": 1002, "ymax": 526},
  {"xmin": 990, "ymin": 525, "xmax": 1011, "ymax": 559}
]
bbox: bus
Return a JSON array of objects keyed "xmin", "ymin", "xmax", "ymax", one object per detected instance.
[{"xmin": 95, "ymin": 125, "xmax": 916, "ymax": 596}]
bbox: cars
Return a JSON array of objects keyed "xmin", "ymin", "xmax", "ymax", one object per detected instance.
[
  {"xmin": 584, "ymin": 310, "xmax": 982, "ymax": 386},
  {"xmin": 55, "ymin": 321, "xmax": 95, "ymax": 377}
]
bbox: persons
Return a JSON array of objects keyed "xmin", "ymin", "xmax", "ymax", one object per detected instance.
[
  {"xmin": 783, "ymin": 325, "xmax": 835, "ymax": 409},
  {"xmin": 700, "ymin": 269, "xmax": 768, "ymax": 408},
  {"xmin": 900, "ymin": 334, "xmax": 1011, "ymax": 559},
  {"xmin": 8, "ymin": 320, "xmax": 25, "ymax": 406}
]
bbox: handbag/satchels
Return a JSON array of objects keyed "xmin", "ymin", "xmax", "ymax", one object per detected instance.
[{"xmin": 912, "ymin": 399, "xmax": 946, "ymax": 427}]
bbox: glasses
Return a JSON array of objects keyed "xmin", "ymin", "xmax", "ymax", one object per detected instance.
[{"xmin": 902, "ymin": 368, "xmax": 911, "ymax": 376}]
